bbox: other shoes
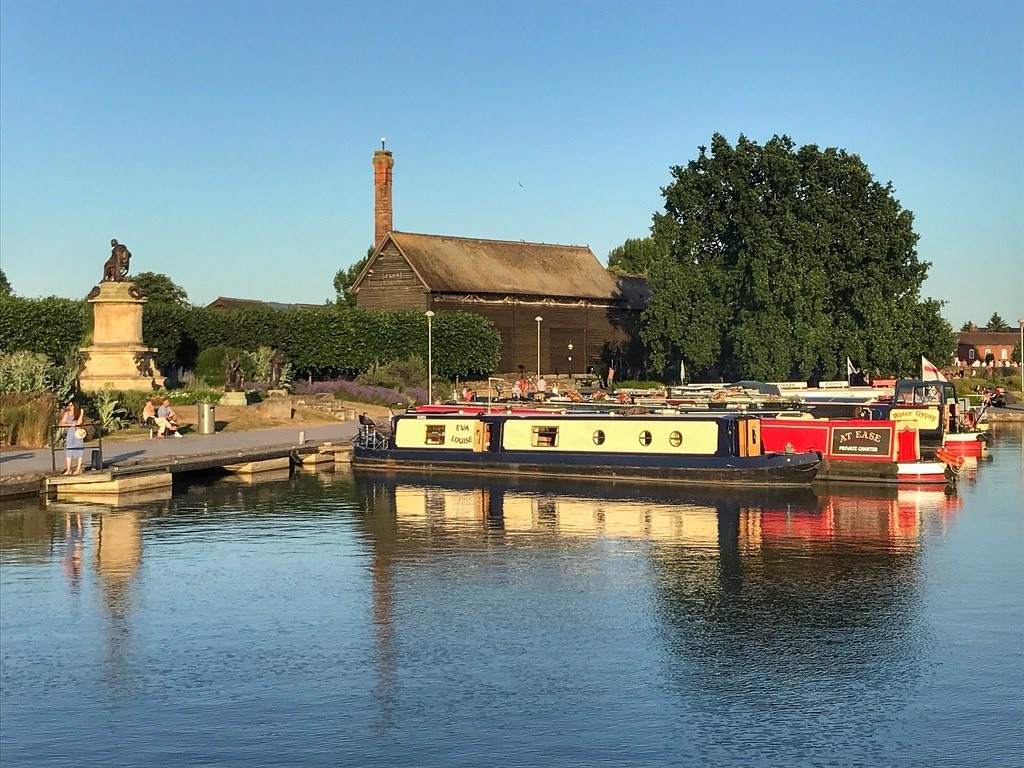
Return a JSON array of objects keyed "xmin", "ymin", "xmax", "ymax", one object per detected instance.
[{"xmin": 156, "ymin": 423, "xmax": 183, "ymax": 439}]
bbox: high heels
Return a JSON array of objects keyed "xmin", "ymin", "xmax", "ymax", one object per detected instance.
[{"xmin": 62, "ymin": 468, "xmax": 82, "ymax": 475}]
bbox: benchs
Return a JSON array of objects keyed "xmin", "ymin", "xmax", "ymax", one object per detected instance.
[{"xmin": 138, "ymin": 414, "xmax": 170, "ymax": 438}]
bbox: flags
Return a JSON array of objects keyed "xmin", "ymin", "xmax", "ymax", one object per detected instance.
[
  {"xmin": 847, "ymin": 358, "xmax": 857, "ymax": 375},
  {"xmin": 922, "ymin": 355, "xmax": 949, "ymax": 383},
  {"xmin": 680, "ymin": 360, "xmax": 686, "ymax": 381}
]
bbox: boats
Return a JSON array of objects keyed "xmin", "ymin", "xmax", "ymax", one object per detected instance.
[
  {"xmin": 347, "ymin": 411, "xmax": 824, "ymax": 491},
  {"xmin": 406, "ymin": 377, "xmax": 995, "ymax": 486}
]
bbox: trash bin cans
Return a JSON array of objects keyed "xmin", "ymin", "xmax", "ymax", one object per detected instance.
[{"xmin": 197, "ymin": 403, "xmax": 215, "ymax": 436}]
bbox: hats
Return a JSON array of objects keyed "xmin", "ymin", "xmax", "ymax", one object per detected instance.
[{"xmin": 75, "ymin": 428, "xmax": 86, "ymax": 439}]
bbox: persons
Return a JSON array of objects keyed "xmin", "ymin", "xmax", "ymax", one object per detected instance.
[
  {"xmin": 954, "ymin": 357, "xmax": 1021, "ymax": 378},
  {"xmin": 974, "ymin": 385, "xmax": 1005, "ymax": 408},
  {"xmin": 511, "ymin": 381, "xmax": 530, "ymax": 403},
  {"xmin": 536, "ymin": 376, "xmax": 547, "ymax": 393},
  {"xmin": 60, "ymin": 400, "xmax": 86, "ymax": 475},
  {"xmin": 552, "ymin": 381, "xmax": 560, "ymax": 396},
  {"xmin": 157, "ymin": 400, "xmax": 183, "ymax": 437},
  {"xmin": 98, "ymin": 239, "xmax": 132, "ymax": 283},
  {"xmin": 462, "ymin": 385, "xmax": 474, "ymax": 402},
  {"xmin": 143, "ymin": 400, "xmax": 178, "ymax": 438}
]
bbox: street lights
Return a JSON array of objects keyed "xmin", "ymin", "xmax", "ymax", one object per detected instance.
[
  {"xmin": 1017, "ymin": 317, "xmax": 1024, "ymax": 392},
  {"xmin": 567, "ymin": 338, "xmax": 574, "ymax": 379},
  {"xmin": 535, "ymin": 316, "xmax": 543, "ymax": 381},
  {"xmin": 424, "ymin": 310, "xmax": 436, "ymax": 405}
]
